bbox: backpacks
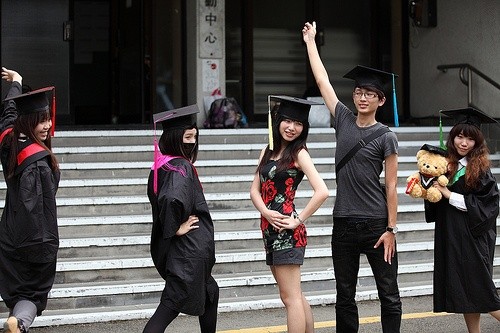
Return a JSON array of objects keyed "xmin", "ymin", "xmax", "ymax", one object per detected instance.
[{"xmin": 203, "ymin": 98, "xmax": 249, "ymax": 129}]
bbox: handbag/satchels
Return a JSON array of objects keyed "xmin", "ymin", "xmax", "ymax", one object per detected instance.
[{"xmin": 306, "ymin": 96, "xmax": 332, "ymax": 128}]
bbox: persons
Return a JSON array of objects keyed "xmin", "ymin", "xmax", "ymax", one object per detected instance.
[
  {"xmin": 302, "ymin": 20, "xmax": 401, "ymax": 333},
  {"xmin": 423, "ymin": 107, "xmax": 500, "ymax": 333},
  {"xmin": 249, "ymin": 95, "xmax": 329, "ymax": 333},
  {"xmin": 143, "ymin": 104, "xmax": 219, "ymax": 333},
  {"xmin": 0, "ymin": 67, "xmax": 61, "ymax": 333}
]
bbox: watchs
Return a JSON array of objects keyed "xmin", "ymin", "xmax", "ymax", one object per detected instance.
[{"xmin": 385, "ymin": 226, "xmax": 398, "ymax": 235}]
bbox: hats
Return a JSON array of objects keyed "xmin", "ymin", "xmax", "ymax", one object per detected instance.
[
  {"xmin": 3, "ymin": 86, "xmax": 56, "ymax": 137},
  {"xmin": 268, "ymin": 94, "xmax": 325, "ymax": 150},
  {"xmin": 342, "ymin": 64, "xmax": 400, "ymax": 128},
  {"xmin": 440, "ymin": 108, "xmax": 499, "ymax": 151},
  {"xmin": 153, "ymin": 104, "xmax": 200, "ymax": 193}
]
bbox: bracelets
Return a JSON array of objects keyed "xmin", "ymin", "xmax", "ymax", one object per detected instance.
[{"xmin": 297, "ymin": 214, "xmax": 301, "ymax": 223}]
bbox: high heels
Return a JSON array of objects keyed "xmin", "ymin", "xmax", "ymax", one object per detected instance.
[{"xmin": 4, "ymin": 316, "xmax": 26, "ymax": 333}]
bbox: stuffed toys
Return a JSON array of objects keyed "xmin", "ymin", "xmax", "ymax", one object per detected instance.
[{"xmin": 406, "ymin": 142, "xmax": 452, "ymax": 203}]
